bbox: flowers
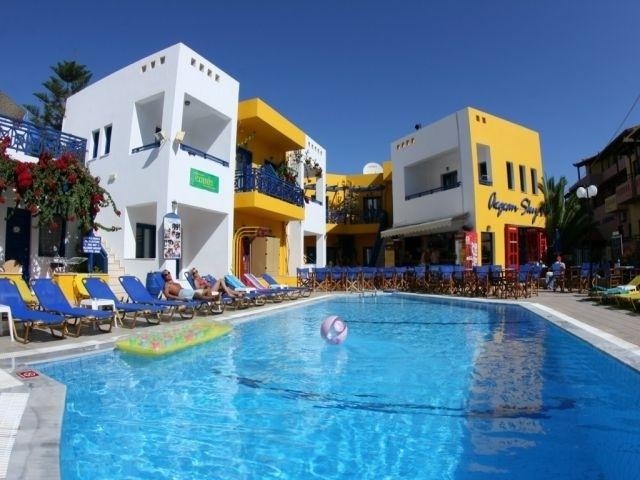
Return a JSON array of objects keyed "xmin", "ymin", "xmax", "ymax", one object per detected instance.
[{"xmin": 0, "ymin": 135, "xmax": 123, "ymax": 235}]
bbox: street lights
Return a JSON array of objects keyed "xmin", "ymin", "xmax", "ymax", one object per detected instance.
[{"xmin": 574, "ymin": 184, "xmax": 599, "ymax": 291}]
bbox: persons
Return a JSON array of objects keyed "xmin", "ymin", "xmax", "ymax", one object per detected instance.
[
  {"xmin": 162, "ymin": 268, "xmax": 242, "ymax": 302},
  {"xmin": 421, "ymin": 247, "xmax": 441, "ymax": 265},
  {"xmin": 599, "ymin": 257, "xmax": 610, "ymax": 271},
  {"xmin": 543, "ymin": 256, "xmax": 565, "ymax": 289}
]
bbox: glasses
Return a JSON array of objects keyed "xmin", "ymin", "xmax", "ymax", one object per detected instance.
[
  {"xmin": 165, "ymin": 272, "xmax": 170, "ymax": 276},
  {"xmin": 193, "ymin": 270, "xmax": 198, "ymax": 276}
]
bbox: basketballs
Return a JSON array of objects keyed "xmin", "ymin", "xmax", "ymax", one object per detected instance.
[{"xmin": 320, "ymin": 315, "xmax": 348, "ymax": 344}]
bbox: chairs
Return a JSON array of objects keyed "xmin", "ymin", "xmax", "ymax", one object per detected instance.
[
  {"xmin": 119, "ymin": 276, "xmax": 197, "ymax": 323},
  {"xmin": 1, "ymin": 277, "xmax": 67, "ymax": 343},
  {"xmin": 546, "ymin": 263, "xmax": 640, "ymax": 313},
  {"xmin": 28, "ymin": 279, "xmax": 117, "ymax": 338},
  {"xmin": 147, "ymin": 270, "xmax": 309, "ymax": 319},
  {"xmin": 82, "ymin": 277, "xmax": 166, "ymax": 329},
  {"xmin": 296, "ymin": 261, "xmax": 541, "ymax": 301}
]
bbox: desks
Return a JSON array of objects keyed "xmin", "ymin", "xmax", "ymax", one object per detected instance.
[
  {"xmin": 1, "ymin": 306, "xmax": 14, "ymax": 343},
  {"xmin": 80, "ymin": 298, "xmax": 117, "ymax": 332}
]
